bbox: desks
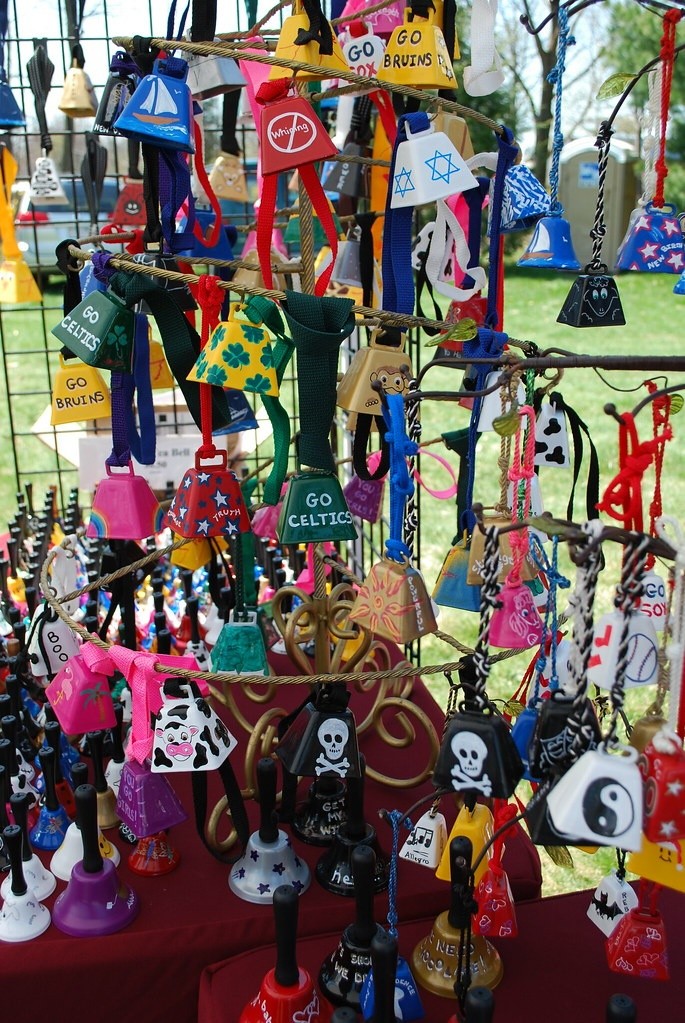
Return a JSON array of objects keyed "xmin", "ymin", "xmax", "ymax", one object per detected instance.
[
  {"xmin": 2, "ymin": 621, "xmax": 544, "ymax": 1023},
  {"xmin": 198, "ymin": 878, "xmax": 685, "ymax": 1023}
]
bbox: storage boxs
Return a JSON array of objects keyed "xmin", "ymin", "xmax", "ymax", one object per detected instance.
[{"xmin": 32, "ymin": 389, "xmax": 293, "ymax": 488}]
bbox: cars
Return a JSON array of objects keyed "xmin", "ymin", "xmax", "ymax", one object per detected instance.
[{"xmin": 11, "ymin": 178, "xmax": 130, "ymax": 290}]
